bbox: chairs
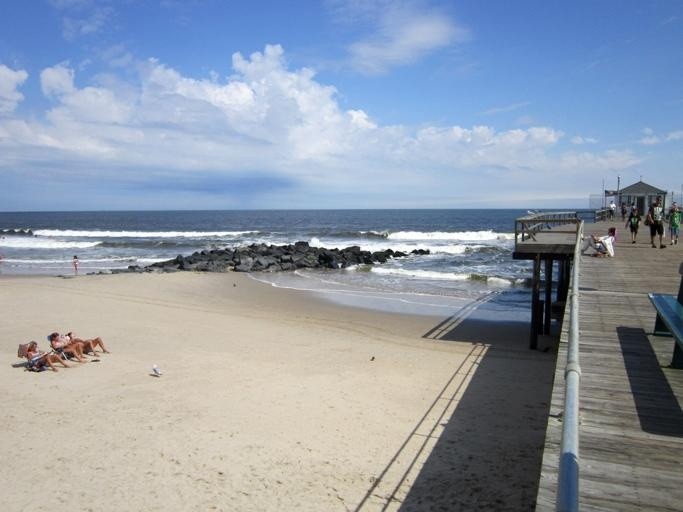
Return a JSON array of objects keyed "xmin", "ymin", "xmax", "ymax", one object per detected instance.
[{"xmin": 20, "ymin": 334, "xmax": 89, "ymax": 372}]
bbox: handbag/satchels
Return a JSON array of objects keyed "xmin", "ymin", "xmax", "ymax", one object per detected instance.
[{"xmin": 644, "ymin": 220, "xmax": 648, "ymax": 225}]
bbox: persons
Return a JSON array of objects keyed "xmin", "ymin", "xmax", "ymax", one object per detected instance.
[
  {"xmin": 580, "ymin": 227, "xmax": 615, "ymax": 256},
  {"xmin": 26, "ymin": 332, "xmax": 111, "ymax": 373},
  {"xmin": 73, "ymin": 255, "xmax": 79, "ymax": 271},
  {"xmin": 607, "ymin": 195, "xmax": 681, "ymax": 249}
]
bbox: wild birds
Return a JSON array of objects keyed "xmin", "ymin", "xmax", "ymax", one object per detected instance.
[
  {"xmin": 527, "ymin": 210, "xmax": 535, "ymax": 217},
  {"xmin": 153, "ymin": 364, "xmax": 163, "ymax": 376}
]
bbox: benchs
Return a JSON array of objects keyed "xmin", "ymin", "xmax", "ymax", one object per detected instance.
[{"xmin": 646, "ymin": 264, "xmax": 683, "ymax": 369}]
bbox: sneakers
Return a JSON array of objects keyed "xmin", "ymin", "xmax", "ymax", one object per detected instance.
[
  {"xmin": 652, "ymin": 244, "xmax": 667, "ymax": 248},
  {"xmin": 670, "ymin": 239, "xmax": 678, "ymax": 245}
]
